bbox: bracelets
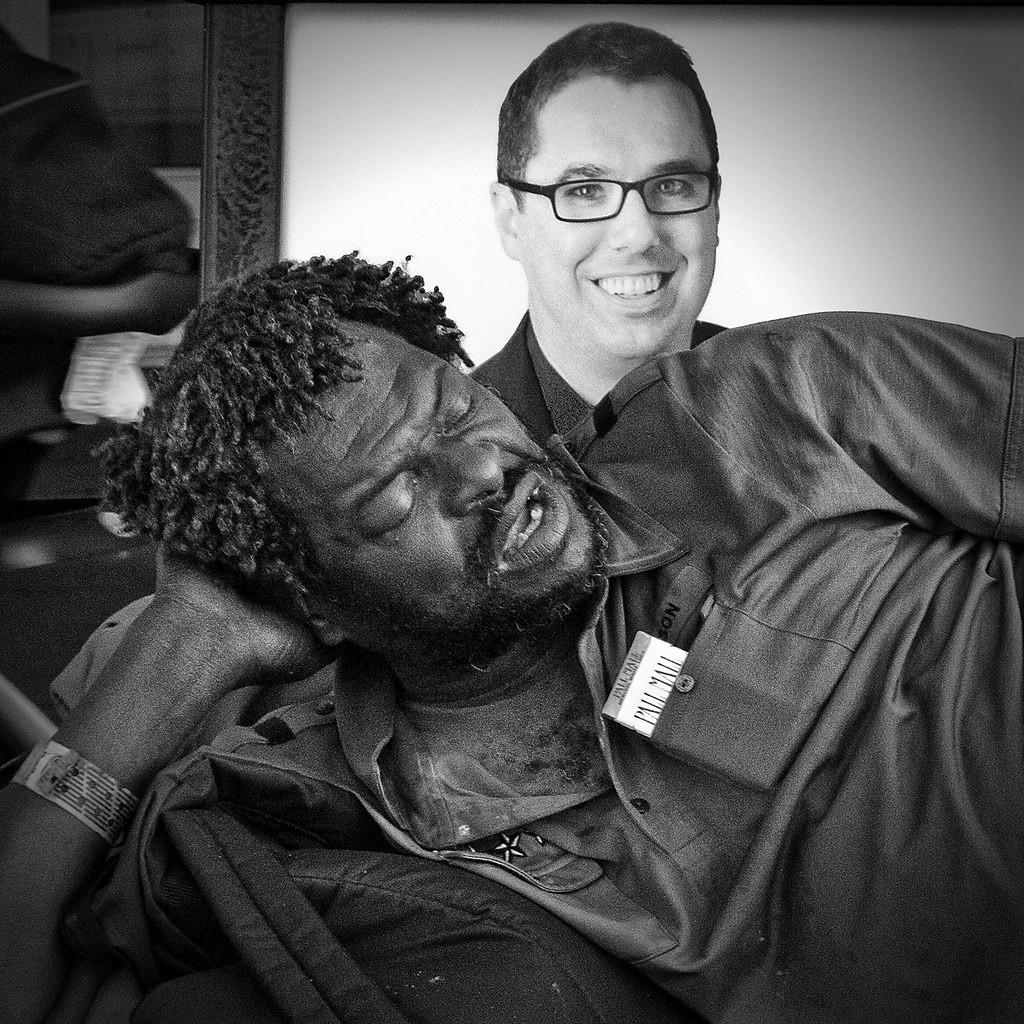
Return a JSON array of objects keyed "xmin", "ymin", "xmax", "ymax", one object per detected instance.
[{"xmin": 12, "ymin": 739, "xmax": 138, "ymax": 846}]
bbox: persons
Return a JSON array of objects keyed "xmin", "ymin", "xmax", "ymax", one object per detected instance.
[
  {"xmin": 0, "ymin": 25, "xmax": 201, "ymax": 334},
  {"xmin": 0, "ymin": 254, "xmax": 1024, "ymax": 1024},
  {"xmin": 464, "ymin": 22, "xmax": 727, "ymax": 451}
]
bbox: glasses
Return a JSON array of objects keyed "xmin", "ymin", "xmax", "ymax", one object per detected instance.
[{"xmin": 501, "ymin": 169, "xmax": 718, "ymax": 223}]
